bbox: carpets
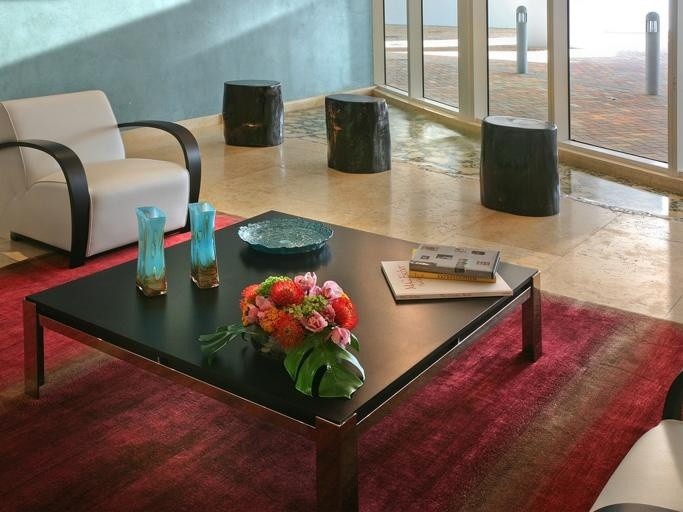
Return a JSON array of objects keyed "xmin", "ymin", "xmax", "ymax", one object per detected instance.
[{"xmin": 0, "ymin": 208, "xmax": 682, "ymax": 512}]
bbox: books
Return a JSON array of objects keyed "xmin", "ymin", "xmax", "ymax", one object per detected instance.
[
  {"xmin": 409, "ymin": 248, "xmax": 497, "ymax": 284},
  {"xmin": 409, "ymin": 244, "xmax": 502, "ymax": 279},
  {"xmin": 380, "ymin": 260, "xmax": 515, "ymax": 301}
]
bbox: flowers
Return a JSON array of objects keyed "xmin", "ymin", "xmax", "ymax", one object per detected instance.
[{"xmin": 196, "ymin": 271, "xmax": 366, "ymax": 402}]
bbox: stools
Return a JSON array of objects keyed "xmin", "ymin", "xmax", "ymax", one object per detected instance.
[
  {"xmin": 222, "ymin": 80, "xmax": 284, "ymax": 147},
  {"xmin": 325, "ymin": 93, "xmax": 392, "ymax": 175},
  {"xmin": 479, "ymin": 115, "xmax": 561, "ymax": 217}
]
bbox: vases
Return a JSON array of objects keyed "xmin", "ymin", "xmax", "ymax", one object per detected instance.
[
  {"xmin": 134, "ymin": 206, "xmax": 169, "ymax": 297},
  {"xmin": 188, "ymin": 201, "xmax": 221, "ymax": 288}
]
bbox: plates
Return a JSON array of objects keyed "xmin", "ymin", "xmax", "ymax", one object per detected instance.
[{"xmin": 237, "ymin": 215, "xmax": 335, "ymax": 254}]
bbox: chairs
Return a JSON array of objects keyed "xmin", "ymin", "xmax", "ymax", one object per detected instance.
[{"xmin": 0, "ymin": 89, "xmax": 203, "ymax": 270}]
